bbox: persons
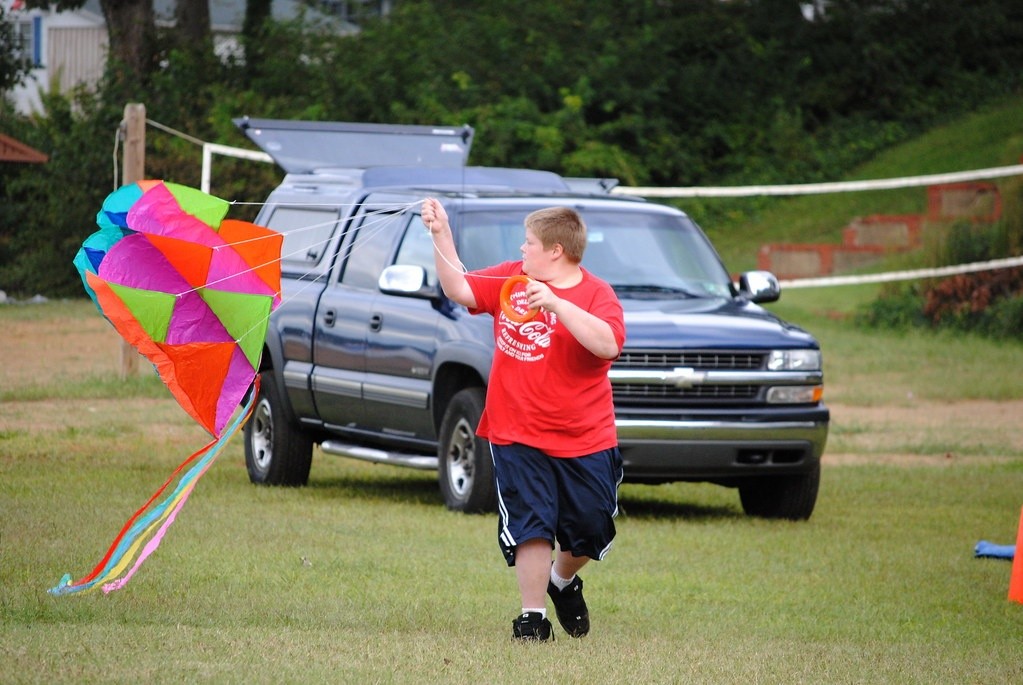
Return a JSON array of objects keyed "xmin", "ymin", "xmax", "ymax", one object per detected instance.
[{"xmin": 420, "ymin": 195, "xmax": 627, "ymax": 646}]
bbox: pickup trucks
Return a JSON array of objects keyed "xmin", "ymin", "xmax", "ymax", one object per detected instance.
[{"xmin": 231, "ymin": 116, "xmax": 830, "ymax": 520}]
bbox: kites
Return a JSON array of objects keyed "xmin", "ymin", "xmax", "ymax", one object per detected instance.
[{"xmin": 45, "ymin": 179, "xmax": 543, "ymax": 592}]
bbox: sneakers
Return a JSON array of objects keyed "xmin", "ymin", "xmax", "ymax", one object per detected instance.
[
  {"xmin": 510, "ymin": 611, "xmax": 554, "ymax": 643},
  {"xmin": 546, "ymin": 560, "xmax": 590, "ymax": 638}
]
